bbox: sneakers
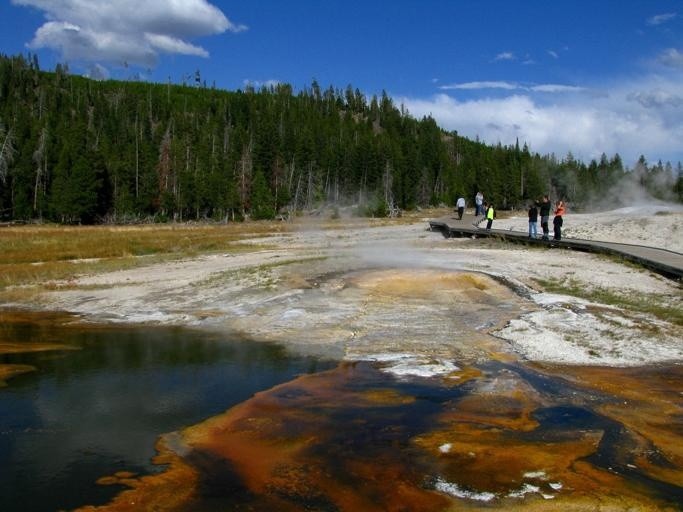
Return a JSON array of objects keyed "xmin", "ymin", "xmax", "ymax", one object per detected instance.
[
  {"xmin": 459, "ymin": 216, "xmax": 462, "ymax": 220},
  {"xmin": 542, "ymin": 235, "xmax": 549, "ymax": 240},
  {"xmin": 486, "ymin": 228, "xmax": 491, "ymax": 231},
  {"xmin": 528, "ymin": 236, "xmax": 537, "ymax": 240},
  {"xmin": 552, "ymin": 237, "xmax": 560, "ymax": 241}
]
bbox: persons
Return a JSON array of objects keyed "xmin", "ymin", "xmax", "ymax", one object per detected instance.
[
  {"xmin": 474, "ymin": 190, "xmax": 483, "ymax": 215},
  {"xmin": 527, "ymin": 202, "xmax": 537, "ymax": 239},
  {"xmin": 456, "ymin": 194, "xmax": 464, "ymax": 219},
  {"xmin": 552, "ymin": 200, "xmax": 564, "ymax": 241},
  {"xmin": 532, "ymin": 195, "xmax": 550, "ymax": 242},
  {"xmin": 485, "ymin": 203, "xmax": 496, "ymax": 231}
]
